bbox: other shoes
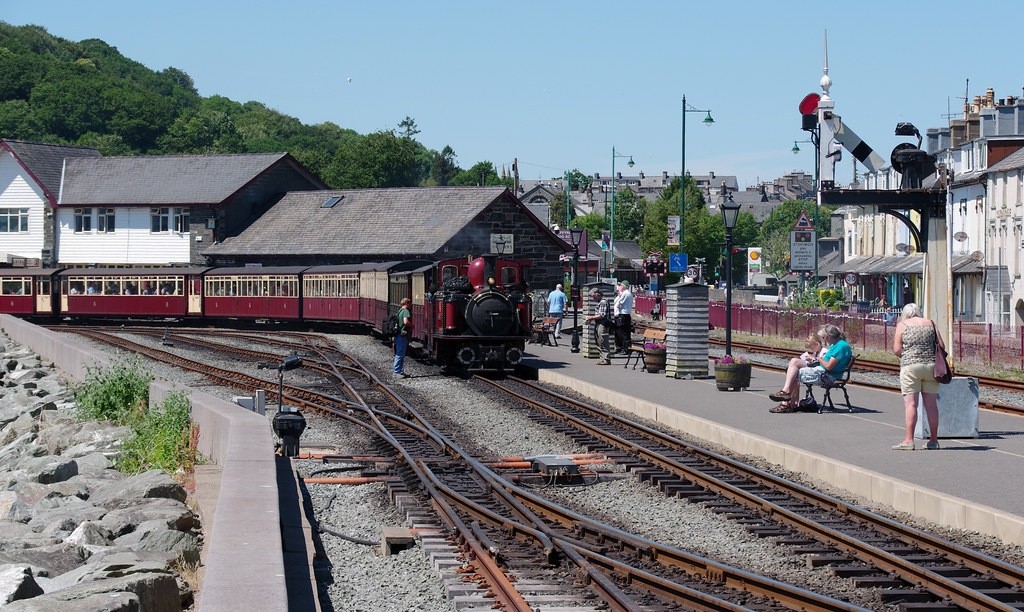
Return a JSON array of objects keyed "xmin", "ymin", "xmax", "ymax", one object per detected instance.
[
  {"xmin": 892, "ymin": 443, "xmax": 915, "ymax": 450},
  {"xmin": 617, "ymin": 349, "xmax": 631, "ymax": 354},
  {"xmin": 393, "ymin": 373, "xmax": 406, "ymax": 379},
  {"xmin": 921, "ymin": 441, "xmax": 939, "ymax": 449},
  {"xmin": 596, "ymin": 358, "xmax": 611, "ymax": 365},
  {"xmin": 555, "ymin": 336, "xmax": 561, "ymax": 339}
]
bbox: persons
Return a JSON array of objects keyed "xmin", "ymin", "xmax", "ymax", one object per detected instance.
[
  {"xmin": 547, "ymin": 283, "xmax": 568, "ymax": 339},
  {"xmin": 882, "ymin": 307, "xmax": 897, "ymax": 326},
  {"xmin": 585, "ymin": 288, "xmax": 611, "ymax": 365},
  {"xmin": 206, "ymin": 281, "xmax": 358, "ymax": 296},
  {"xmin": 769, "ymin": 324, "xmax": 853, "ymax": 413},
  {"xmin": 70, "ymin": 282, "xmax": 183, "ymax": 295},
  {"xmin": 853, "ymin": 291, "xmax": 857, "ymax": 302},
  {"xmin": 891, "ymin": 303, "xmax": 945, "ymax": 450},
  {"xmin": 879, "ymin": 295, "xmax": 888, "ymax": 311},
  {"xmin": 394, "ymin": 297, "xmax": 415, "ymax": 375},
  {"xmin": 2, "ymin": 283, "xmax": 22, "ymax": 294},
  {"xmin": 612, "ymin": 280, "xmax": 632, "ymax": 354}
]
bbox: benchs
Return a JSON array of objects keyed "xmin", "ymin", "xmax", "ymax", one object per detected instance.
[
  {"xmin": 804, "ymin": 354, "xmax": 861, "ymax": 413},
  {"xmin": 625, "ymin": 329, "xmax": 668, "ymax": 372},
  {"xmin": 534, "ymin": 317, "xmax": 560, "ymax": 347}
]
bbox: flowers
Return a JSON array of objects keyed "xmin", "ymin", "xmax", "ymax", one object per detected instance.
[
  {"xmin": 643, "ymin": 341, "xmax": 667, "ymax": 350},
  {"xmin": 715, "ymin": 354, "xmax": 750, "ymax": 363}
]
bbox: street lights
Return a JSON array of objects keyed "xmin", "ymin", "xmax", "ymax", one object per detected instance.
[
  {"xmin": 568, "ymin": 221, "xmax": 590, "ymax": 353},
  {"xmin": 718, "ymin": 190, "xmax": 742, "ymax": 355},
  {"xmin": 566, "ymin": 172, "xmax": 590, "ymax": 230},
  {"xmin": 610, "ymin": 146, "xmax": 636, "ymax": 279},
  {"xmin": 790, "ymin": 134, "xmax": 820, "ymax": 308},
  {"xmin": 681, "ymin": 94, "xmax": 716, "ymax": 283}
]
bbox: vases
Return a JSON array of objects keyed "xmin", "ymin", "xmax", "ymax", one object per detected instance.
[
  {"xmin": 715, "ymin": 365, "xmax": 751, "ymax": 392},
  {"xmin": 644, "ymin": 350, "xmax": 666, "ymax": 373}
]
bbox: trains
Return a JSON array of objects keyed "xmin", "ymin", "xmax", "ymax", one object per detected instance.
[{"xmin": 0, "ymin": 253, "xmax": 535, "ymax": 377}]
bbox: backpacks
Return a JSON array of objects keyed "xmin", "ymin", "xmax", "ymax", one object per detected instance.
[{"xmin": 382, "ymin": 309, "xmax": 406, "ymax": 337}]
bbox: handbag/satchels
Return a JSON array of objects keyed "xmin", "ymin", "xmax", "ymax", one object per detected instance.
[
  {"xmin": 608, "ymin": 321, "xmax": 615, "ymax": 335},
  {"xmin": 807, "ymin": 362, "xmax": 820, "ymax": 367},
  {"xmin": 931, "ymin": 320, "xmax": 952, "ymax": 384},
  {"xmin": 800, "ymin": 397, "xmax": 818, "ymax": 413}
]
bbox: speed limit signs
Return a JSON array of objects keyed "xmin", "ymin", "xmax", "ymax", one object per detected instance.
[
  {"xmin": 845, "ymin": 272, "xmax": 858, "ymax": 285},
  {"xmin": 686, "ymin": 266, "xmax": 699, "ymax": 279}
]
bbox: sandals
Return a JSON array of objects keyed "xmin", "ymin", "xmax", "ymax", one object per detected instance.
[
  {"xmin": 769, "ymin": 400, "xmax": 794, "ymax": 413},
  {"xmin": 769, "ymin": 390, "xmax": 791, "ymax": 402}
]
